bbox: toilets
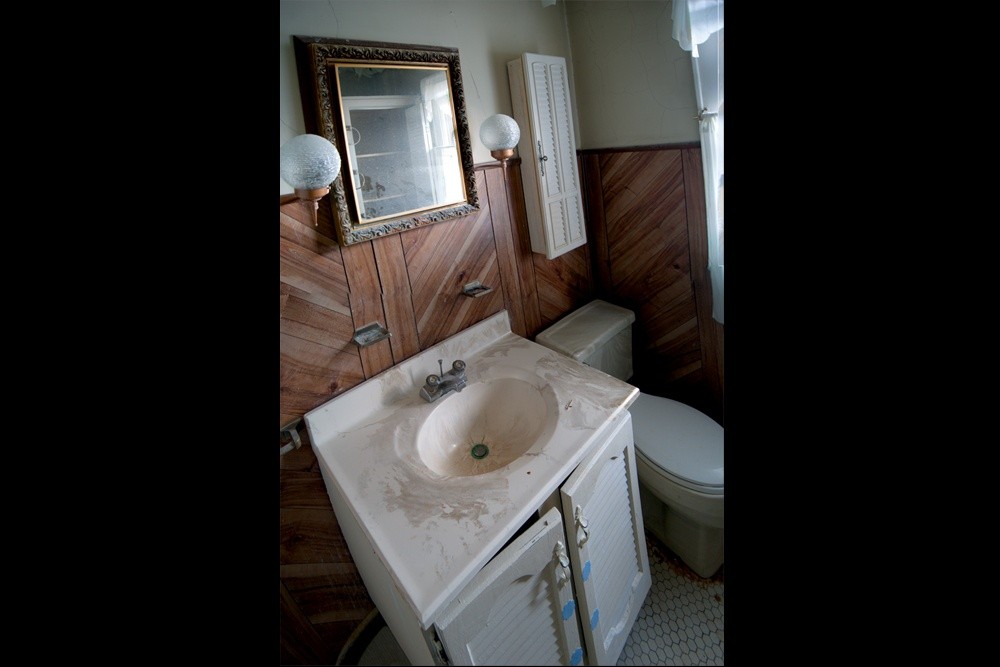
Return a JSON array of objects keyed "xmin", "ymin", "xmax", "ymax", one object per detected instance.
[{"xmin": 538, "ymin": 301, "xmax": 725, "ymax": 580}]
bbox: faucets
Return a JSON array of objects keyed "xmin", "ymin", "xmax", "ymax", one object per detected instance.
[{"xmin": 419, "ymin": 358, "xmax": 468, "ymax": 403}]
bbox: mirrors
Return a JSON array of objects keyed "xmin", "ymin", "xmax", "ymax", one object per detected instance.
[{"xmin": 293, "ymin": 34, "xmax": 481, "ymax": 247}]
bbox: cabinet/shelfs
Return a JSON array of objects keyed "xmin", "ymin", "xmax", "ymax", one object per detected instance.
[
  {"xmin": 507, "ymin": 53, "xmax": 588, "ymax": 260},
  {"xmin": 341, "ymin": 94, "xmax": 437, "ymax": 220},
  {"xmin": 316, "ymin": 410, "xmax": 652, "ymax": 667}
]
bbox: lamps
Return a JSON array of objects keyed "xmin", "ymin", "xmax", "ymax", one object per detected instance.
[
  {"xmin": 280, "ymin": 134, "xmax": 341, "ymax": 226},
  {"xmin": 474, "ymin": 114, "xmax": 520, "ymax": 189}
]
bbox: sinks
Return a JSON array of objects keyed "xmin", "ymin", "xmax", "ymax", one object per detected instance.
[{"xmin": 392, "ymin": 364, "xmax": 560, "ymax": 490}]
